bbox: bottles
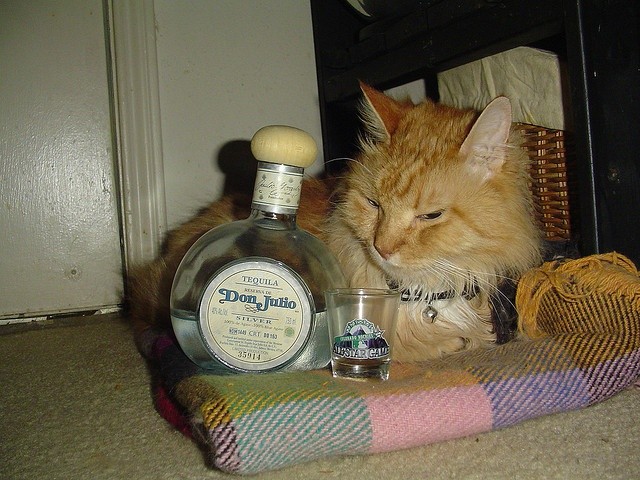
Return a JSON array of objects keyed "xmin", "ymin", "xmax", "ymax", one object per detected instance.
[{"xmin": 171, "ymin": 126, "xmax": 350, "ymax": 376}]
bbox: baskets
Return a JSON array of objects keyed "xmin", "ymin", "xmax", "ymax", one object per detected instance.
[{"xmin": 382, "ymin": 46, "xmax": 573, "ymax": 244}]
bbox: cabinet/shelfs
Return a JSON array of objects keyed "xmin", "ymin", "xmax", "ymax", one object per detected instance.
[{"xmin": 308, "ymin": 1, "xmax": 639, "ymax": 268}]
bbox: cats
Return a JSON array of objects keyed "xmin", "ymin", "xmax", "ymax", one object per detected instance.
[{"xmin": 112, "ymin": 77, "xmax": 555, "ymax": 382}]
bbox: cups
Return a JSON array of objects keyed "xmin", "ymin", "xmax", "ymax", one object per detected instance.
[{"xmin": 326, "ymin": 289, "xmax": 400, "ymax": 383}]
